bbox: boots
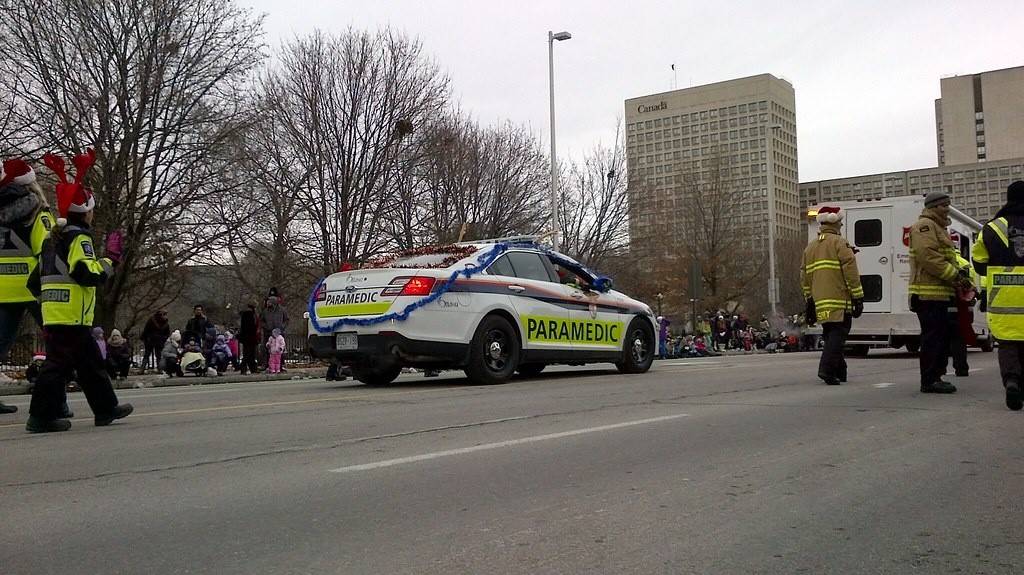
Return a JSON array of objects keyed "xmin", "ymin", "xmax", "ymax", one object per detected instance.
[{"xmin": 719, "ymin": 344, "xmax": 725, "ymax": 352}]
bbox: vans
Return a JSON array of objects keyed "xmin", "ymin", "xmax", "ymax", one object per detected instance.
[{"xmin": 804, "ymin": 191, "xmax": 997, "ymax": 356}]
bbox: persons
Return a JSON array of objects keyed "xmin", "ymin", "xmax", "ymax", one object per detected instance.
[
  {"xmin": 26, "ymin": 183, "xmax": 132, "ymax": 431},
  {"xmin": 970, "ymin": 179, "xmax": 1024, "ymax": 410},
  {"xmin": 799, "ymin": 207, "xmax": 863, "ymax": 386},
  {"xmin": 908, "ymin": 191, "xmax": 980, "ymax": 392},
  {"xmin": 0, "ymin": 160, "xmax": 75, "ymax": 419},
  {"xmin": 92, "ymin": 327, "xmax": 132, "ymax": 381},
  {"xmin": 560, "ymin": 275, "xmax": 581, "ymax": 289},
  {"xmin": 710, "ymin": 309, "xmax": 770, "ymax": 349},
  {"xmin": 26, "ymin": 352, "xmax": 47, "ymax": 392},
  {"xmin": 656, "ymin": 315, "xmax": 719, "ymax": 356},
  {"xmin": 139, "ymin": 307, "xmax": 170, "ymax": 375},
  {"xmin": 238, "ymin": 287, "xmax": 290, "ymax": 374},
  {"xmin": 161, "ymin": 304, "xmax": 238, "ymax": 376}
]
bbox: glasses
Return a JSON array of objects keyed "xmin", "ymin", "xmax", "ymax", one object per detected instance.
[{"xmin": 936, "ymin": 202, "xmax": 949, "ymax": 207}]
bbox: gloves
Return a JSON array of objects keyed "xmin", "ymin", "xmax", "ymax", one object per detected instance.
[
  {"xmin": 104, "ymin": 232, "xmax": 122, "ymax": 264},
  {"xmin": 720, "ymin": 332, "xmax": 725, "ymax": 336},
  {"xmin": 851, "ymin": 300, "xmax": 864, "ymax": 318},
  {"xmin": 804, "ymin": 302, "xmax": 817, "ymax": 325},
  {"xmin": 977, "ymin": 290, "xmax": 987, "ymax": 313}
]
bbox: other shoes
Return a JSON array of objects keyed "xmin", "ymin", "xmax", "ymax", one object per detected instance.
[
  {"xmin": 817, "ymin": 371, "xmax": 840, "ymax": 385},
  {"xmin": 921, "ymin": 380, "xmax": 956, "ymax": 393},
  {"xmin": 955, "ymin": 369, "xmax": 969, "ymax": 377},
  {"xmin": 1005, "ymin": 382, "xmax": 1023, "ymax": 410},
  {"xmin": 0, "ymin": 403, "xmax": 133, "ymax": 437},
  {"xmin": 326, "ymin": 371, "xmax": 347, "ymax": 382},
  {"xmin": 424, "ymin": 370, "xmax": 439, "ymax": 377},
  {"xmin": 236, "ymin": 365, "xmax": 287, "ymax": 375},
  {"xmin": 838, "ymin": 368, "xmax": 847, "ymax": 382}
]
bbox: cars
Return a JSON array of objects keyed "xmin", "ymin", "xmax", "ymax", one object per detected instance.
[{"xmin": 304, "ymin": 238, "xmax": 659, "ymax": 385}]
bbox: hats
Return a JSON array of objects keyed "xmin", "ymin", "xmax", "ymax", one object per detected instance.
[
  {"xmin": 816, "ymin": 207, "xmax": 844, "ymax": 223},
  {"xmin": 93, "ymin": 327, "xmax": 104, "ymax": 336},
  {"xmin": 1007, "ymin": 181, "xmax": 1024, "ymax": 202},
  {"xmin": 925, "ymin": 191, "xmax": 951, "ymax": 208},
  {"xmin": 272, "ymin": 328, "xmax": 281, "ymax": 337},
  {"xmin": 0, "ymin": 159, "xmax": 36, "ymax": 185},
  {"xmin": 657, "ymin": 316, "xmax": 662, "ymax": 323},
  {"xmin": 33, "ymin": 352, "xmax": 46, "ymax": 361},
  {"xmin": 111, "ymin": 329, "xmax": 120, "ymax": 339},
  {"xmin": 45, "ymin": 147, "xmax": 95, "ymax": 226},
  {"xmin": 733, "ymin": 316, "xmax": 738, "ymax": 319},
  {"xmin": 171, "ymin": 328, "xmax": 235, "ymax": 342}
]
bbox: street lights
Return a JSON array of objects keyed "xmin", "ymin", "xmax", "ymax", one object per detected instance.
[
  {"xmin": 545, "ymin": 31, "xmax": 574, "ymax": 281},
  {"xmin": 689, "ymin": 297, "xmax": 700, "ymax": 341}
]
